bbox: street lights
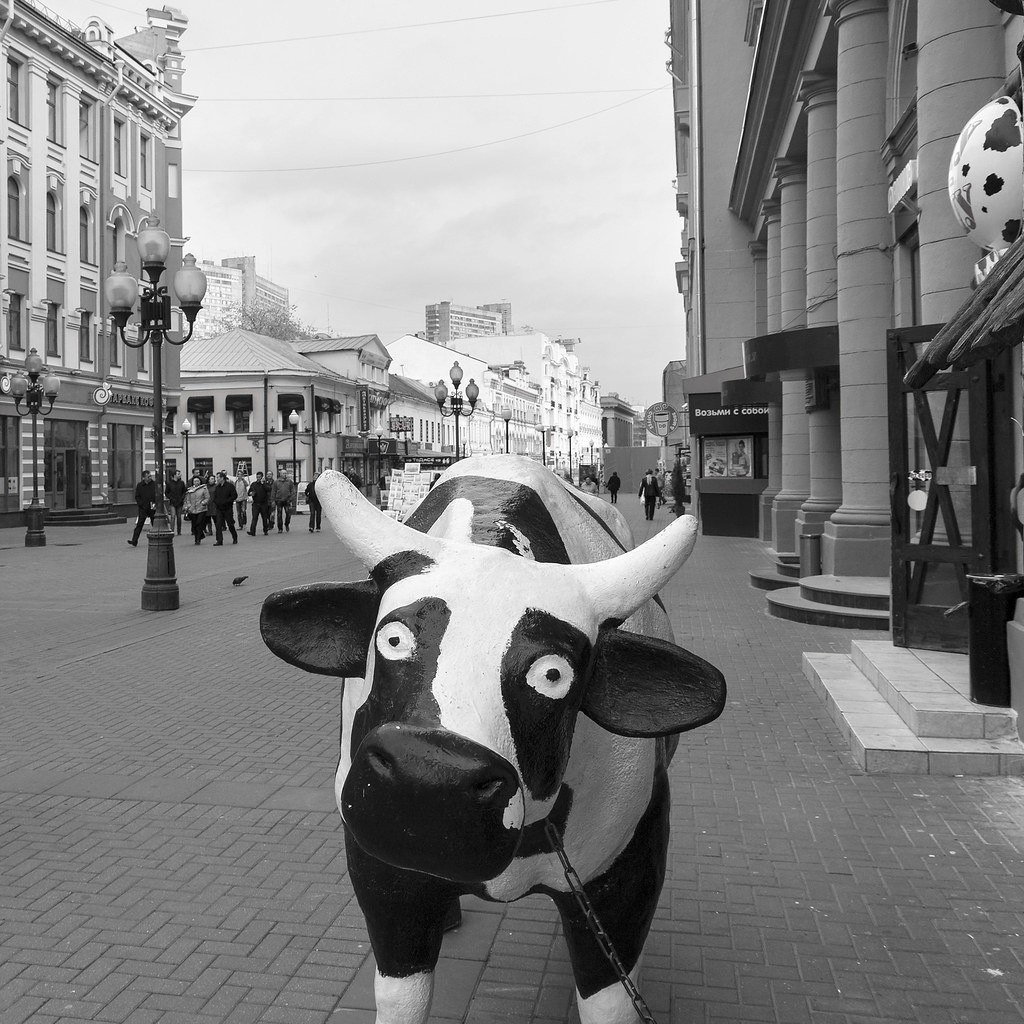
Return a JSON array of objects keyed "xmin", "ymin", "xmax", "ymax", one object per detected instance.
[
  {"xmin": 502, "ymin": 407, "xmax": 512, "ymax": 454},
  {"xmin": 567, "ymin": 428, "xmax": 573, "ymax": 478},
  {"xmin": 589, "ymin": 439, "xmax": 594, "ymax": 464},
  {"xmin": 9, "ymin": 346, "xmax": 61, "ymax": 547},
  {"xmin": 182, "ymin": 419, "xmax": 192, "ymax": 521},
  {"xmin": 540, "ymin": 425, "xmax": 547, "ymax": 466},
  {"xmin": 461, "ymin": 438, "xmax": 468, "ymax": 459},
  {"xmin": 375, "ymin": 425, "xmax": 384, "ymax": 505},
  {"xmin": 289, "ymin": 408, "xmax": 300, "ymax": 515},
  {"xmin": 433, "ymin": 360, "xmax": 479, "ymax": 462},
  {"xmin": 101, "ymin": 207, "xmax": 208, "ymax": 610}
]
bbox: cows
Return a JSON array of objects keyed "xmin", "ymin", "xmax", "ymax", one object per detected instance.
[{"xmin": 259, "ymin": 451, "xmax": 729, "ymax": 1023}]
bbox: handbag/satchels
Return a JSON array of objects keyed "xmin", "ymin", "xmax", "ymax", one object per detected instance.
[
  {"xmin": 640, "ymin": 487, "xmax": 646, "ymax": 505},
  {"xmin": 184, "ymin": 509, "xmax": 192, "ymax": 521}
]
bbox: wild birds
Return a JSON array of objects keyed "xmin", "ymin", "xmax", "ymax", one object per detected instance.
[{"xmin": 233, "ymin": 576, "xmax": 249, "ymax": 586}]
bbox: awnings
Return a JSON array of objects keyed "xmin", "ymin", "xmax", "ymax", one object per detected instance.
[
  {"xmin": 315, "ymin": 395, "xmax": 341, "ymax": 414},
  {"xmin": 187, "ymin": 396, "xmax": 214, "ymax": 413},
  {"xmin": 226, "ymin": 394, "xmax": 253, "ymax": 411},
  {"xmin": 278, "ymin": 394, "xmax": 304, "ymax": 411}
]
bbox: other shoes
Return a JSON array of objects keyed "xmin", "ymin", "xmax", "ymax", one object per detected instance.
[
  {"xmin": 127, "ymin": 540, "xmax": 137, "ymax": 546},
  {"xmin": 247, "ymin": 531, "xmax": 255, "ymax": 536},
  {"xmin": 264, "ymin": 531, "xmax": 268, "ymax": 535},
  {"xmin": 213, "ymin": 541, "xmax": 223, "ymax": 546},
  {"xmin": 316, "ymin": 529, "xmax": 320, "ymax": 532},
  {"xmin": 309, "ymin": 527, "xmax": 314, "ymax": 533},
  {"xmin": 233, "ymin": 537, "xmax": 237, "ymax": 544}
]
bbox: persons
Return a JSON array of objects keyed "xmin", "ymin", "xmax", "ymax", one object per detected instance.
[
  {"xmin": 165, "ymin": 470, "xmax": 188, "ymax": 535},
  {"xmin": 186, "ymin": 468, "xmax": 250, "ymax": 536},
  {"xmin": 557, "ymin": 473, "xmax": 599, "ymax": 496},
  {"xmin": 343, "ymin": 467, "xmax": 362, "ymax": 490},
  {"xmin": 606, "ymin": 472, "xmax": 620, "ymax": 503},
  {"xmin": 212, "ymin": 472, "xmax": 238, "ymax": 546},
  {"xmin": 183, "ymin": 476, "xmax": 211, "ymax": 545},
  {"xmin": 266, "ymin": 470, "xmax": 276, "ymax": 530},
  {"xmin": 270, "ymin": 470, "xmax": 296, "ymax": 533},
  {"xmin": 247, "ymin": 472, "xmax": 270, "ymax": 536},
  {"xmin": 376, "ymin": 471, "xmax": 389, "ymax": 490},
  {"xmin": 305, "ymin": 472, "xmax": 322, "ymax": 533},
  {"xmin": 429, "ymin": 473, "xmax": 441, "ymax": 490},
  {"xmin": 639, "ymin": 468, "xmax": 668, "ymax": 520},
  {"xmin": 737, "ymin": 440, "xmax": 750, "ymax": 476},
  {"xmin": 127, "ymin": 470, "xmax": 156, "ymax": 547}
]
